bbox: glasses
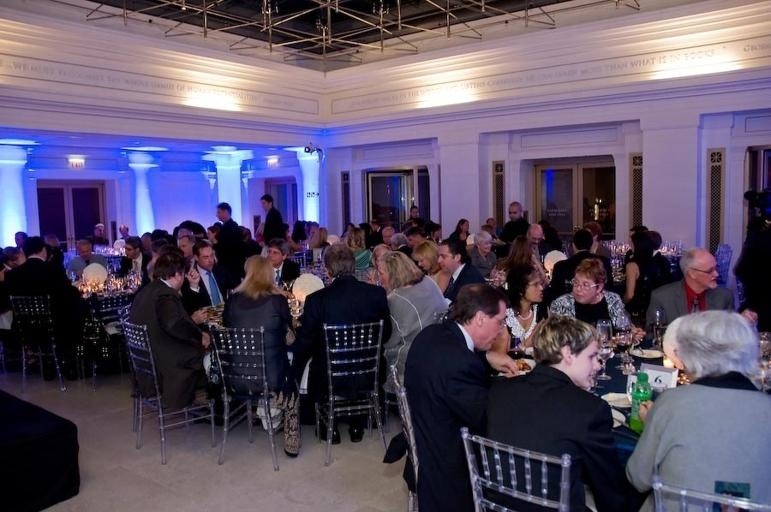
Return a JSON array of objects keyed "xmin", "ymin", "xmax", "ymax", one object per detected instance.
[
  {"xmin": 572, "ymin": 280, "xmax": 598, "ymax": 289},
  {"xmin": 691, "ymin": 264, "xmax": 717, "ymax": 273}
]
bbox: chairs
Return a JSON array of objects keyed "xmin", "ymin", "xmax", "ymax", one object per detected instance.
[
  {"xmin": 81, "ymin": 293, "xmax": 136, "ymax": 390},
  {"xmin": 390, "ymin": 365, "xmax": 421, "ymax": 510},
  {"xmin": 715, "ymin": 244, "xmax": 734, "ymax": 287},
  {"xmin": 461, "ymin": 427, "xmax": 572, "ymax": 511},
  {"xmin": 320, "ymin": 319, "xmax": 392, "ymax": 467},
  {"xmin": 119, "ymin": 318, "xmax": 216, "ymax": 465},
  {"xmin": 9, "ymin": 295, "xmax": 73, "ymax": 391},
  {"xmin": 286, "ymin": 255, "xmax": 307, "ymax": 269},
  {"xmin": 651, "ymin": 475, "xmax": 769, "ymax": 511},
  {"xmin": 433, "ymin": 310, "xmax": 448, "ymax": 326},
  {"xmin": 208, "ymin": 319, "xmax": 279, "ymax": 470}
]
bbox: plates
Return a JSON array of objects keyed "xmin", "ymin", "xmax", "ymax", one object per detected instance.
[
  {"xmin": 631, "ymin": 350, "xmax": 665, "ymax": 358},
  {"xmin": 495, "ymin": 359, "xmax": 536, "ymax": 378},
  {"xmin": 600, "ymin": 392, "xmax": 631, "ymax": 408}
]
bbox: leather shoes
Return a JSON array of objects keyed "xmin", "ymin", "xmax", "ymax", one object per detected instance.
[
  {"xmin": 347, "ymin": 424, "xmax": 363, "ymax": 442},
  {"xmin": 316, "ymin": 423, "xmax": 341, "ymax": 443}
]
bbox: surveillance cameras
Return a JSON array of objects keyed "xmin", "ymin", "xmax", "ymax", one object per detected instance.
[{"xmin": 305, "ymin": 145, "xmax": 316, "ymax": 152}]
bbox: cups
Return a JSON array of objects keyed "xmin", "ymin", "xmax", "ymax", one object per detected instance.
[
  {"xmin": 749, "ymin": 331, "xmax": 771, "ymax": 390},
  {"xmin": 506, "ymin": 334, "xmax": 526, "ymax": 360},
  {"xmin": 625, "ymin": 374, "xmax": 638, "ymax": 404},
  {"xmin": 660, "ymin": 238, "xmax": 684, "ymax": 262},
  {"xmin": 663, "ymin": 353, "xmax": 675, "ymax": 369}
]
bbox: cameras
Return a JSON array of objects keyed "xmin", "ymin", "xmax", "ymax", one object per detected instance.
[{"xmin": 744, "ymin": 191, "xmax": 767, "ymax": 209}]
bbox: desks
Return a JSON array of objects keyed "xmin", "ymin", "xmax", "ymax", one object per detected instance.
[{"xmin": 485, "ymin": 327, "xmax": 771, "ymax": 461}]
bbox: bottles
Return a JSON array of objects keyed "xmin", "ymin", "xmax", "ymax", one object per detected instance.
[
  {"xmin": 631, "ymin": 373, "xmax": 654, "ymax": 431},
  {"xmin": 653, "ymin": 309, "xmax": 663, "ymax": 346}
]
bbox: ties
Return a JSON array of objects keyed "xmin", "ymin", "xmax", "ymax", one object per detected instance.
[
  {"xmin": 275, "ymin": 269, "xmax": 280, "ymax": 281},
  {"xmin": 447, "ymin": 274, "xmax": 453, "ymax": 285},
  {"xmin": 132, "ymin": 261, "xmax": 139, "ymax": 271},
  {"xmin": 206, "ymin": 272, "xmax": 222, "ymax": 305}
]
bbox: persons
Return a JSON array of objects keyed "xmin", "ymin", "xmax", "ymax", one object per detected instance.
[
  {"xmin": 493, "ymin": 219, "xmax": 670, "ymax": 361},
  {"xmin": 643, "ymin": 248, "xmax": 760, "ymax": 340},
  {"xmin": 402, "ymin": 283, "xmax": 521, "ymax": 512},
  {"xmin": 624, "ymin": 308, "xmax": 770, "ymax": 511},
  {"xmin": 2, "ymin": 203, "xmax": 264, "ymax": 428},
  {"xmin": 469, "ymin": 313, "xmax": 654, "ymax": 512},
  {"xmin": 222, "ymin": 194, "xmax": 531, "ymax": 459}
]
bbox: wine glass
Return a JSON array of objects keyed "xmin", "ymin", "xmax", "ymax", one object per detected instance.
[
  {"xmin": 615, "ymin": 311, "xmax": 636, "ymax": 376},
  {"xmin": 601, "ymin": 239, "xmax": 635, "ymax": 272},
  {"xmin": 65, "ymin": 239, "xmax": 140, "ymax": 298},
  {"xmin": 596, "ymin": 320, "xmax": 614, "ymax": 381}
]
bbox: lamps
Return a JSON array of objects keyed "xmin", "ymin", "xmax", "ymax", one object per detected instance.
[
  {"xmin": 264, "ymin": 154, "xmax": 283, "ymax": 169},
  {"xmin": 65, "ymin": 155, "xmax": 88, "ymax": 168}
]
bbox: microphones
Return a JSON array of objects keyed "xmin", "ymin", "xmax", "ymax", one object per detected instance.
[{"xmin": 85, "ymin": 259, "xmax": 90, "ymax": 265}]
